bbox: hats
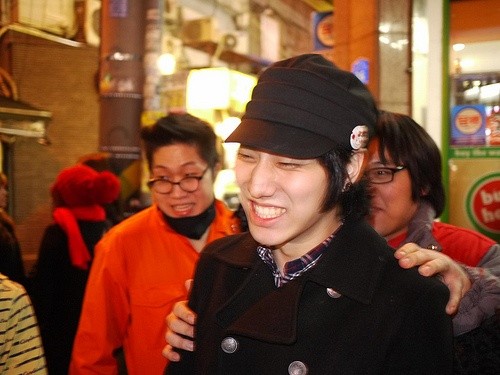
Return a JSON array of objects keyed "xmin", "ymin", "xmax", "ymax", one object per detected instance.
[
  {"xmin": 56, "ymin": 165, "xmax": 120, "ymax": 209},
  {"xmin": 225, "ymin": 53, "xmax": 377, "ymax": 158}
]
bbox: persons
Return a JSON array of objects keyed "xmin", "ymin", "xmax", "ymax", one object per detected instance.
[
  {"xmin": 163, "ymin": 111, "xmax": 500, "ymax": 375},
  {"xmin": 68, "ymin": 112, "xmax": 250, "ymax": 375},
  {"xmin": 0, "ymin": 172, "xmax": 24, "ymax": 280},
  {"xmin": 163, "ymin": 53, "xmax": 454, "ymax": 375},
  {"xmin": 26, "ymin": 164, "xmax": 122, "ymax": 375}
]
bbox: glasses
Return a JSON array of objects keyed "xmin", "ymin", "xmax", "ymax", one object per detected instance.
[
  {"xmin": 146, "ymin": 164, "xmax": 209, "ymax": 194},
  {"xmin": 364, "ymin": 165, "xmax": 408, "ymax": 184}
]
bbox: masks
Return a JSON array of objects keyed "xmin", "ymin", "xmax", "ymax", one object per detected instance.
[{"xmin": 163, "ymin": 202, "xmax": 214, "ymax": 239}]
